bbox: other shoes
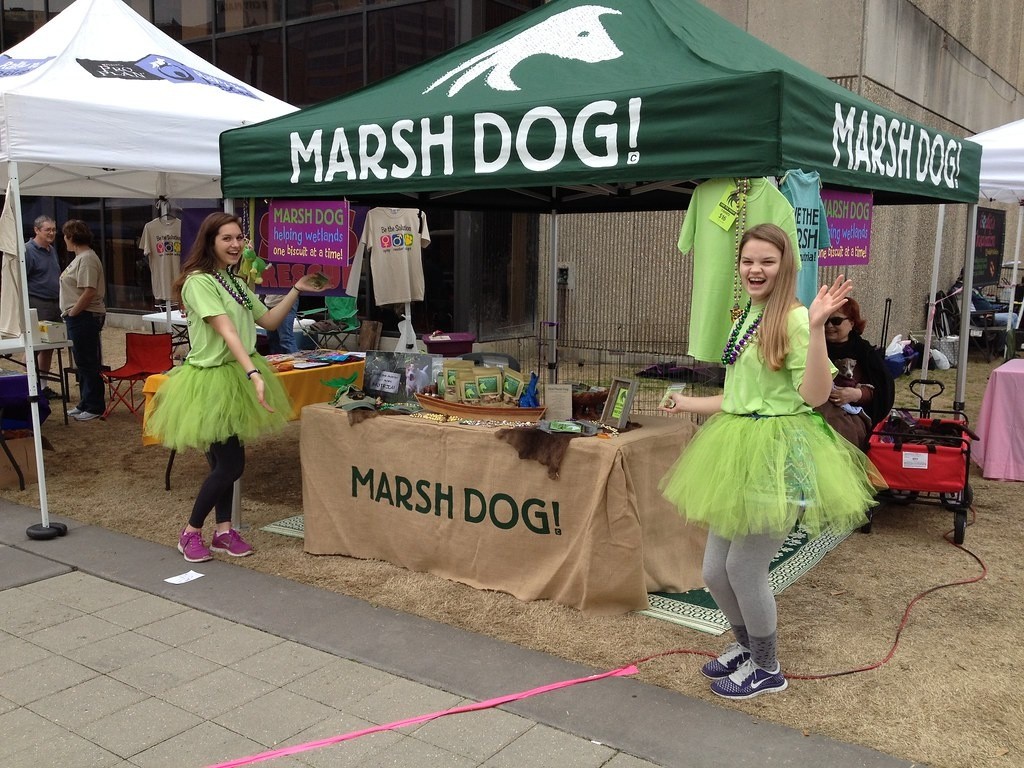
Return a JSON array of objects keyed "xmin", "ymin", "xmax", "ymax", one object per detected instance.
[
  {"xmin": 993, "ymin": 349, "xmax": 1004, "ymax": 357},
  {"xmin": 41, "ymin": 386, "xmax": 62, "ymax": 398}
]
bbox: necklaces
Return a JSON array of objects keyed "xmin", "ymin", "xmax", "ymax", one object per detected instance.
[
  {"xmin": 730, "ymin": 176, "xmax": 747, "ymax": 324},
  {"xmin": 720, "ymin": 298, "xmax": 765, "ymax": 365},
  {"xmin": 211, "ymin": 263, "xmax": 253, "ymax": 311}
]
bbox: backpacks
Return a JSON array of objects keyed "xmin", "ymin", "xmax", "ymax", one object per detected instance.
[{"xmin": 926, "ymin": 290, "xmax": 960, "ymax": 336}]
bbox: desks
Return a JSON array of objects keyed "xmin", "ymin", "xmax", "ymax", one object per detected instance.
[
  {"xmin": 142, "ymin": 310, "xmax": 192, "ymax": 361},
  {"xmin": 142, "ymin": 349, "xmax": 366, "ymax": 491},
  {"xmin": 299, "ymin": 400, "xmax": 709, "ymax": 614},
  {"xmin": 0, "ymin": 333, "xmax": 73, "ymax": 425},
  {"xmin": 969, "ymin": 359, "xmax": 1024, "ymax": 482}
]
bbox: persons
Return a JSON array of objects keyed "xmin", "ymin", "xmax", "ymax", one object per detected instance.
[
  {"xmin": 59, "ymin": 224, "xmax": 107, "ymax": 421},
  {"xmin": 814, "ymin": 267, "xmax": 1023, "ymax": 445},
  {"xmin": 264, "ymin": 294, "xmax": 299, "ymax": 355},
  {"xmin": 218, "ymin": 0, "xmax": 985, "ymax": 512},
  {"xmin": 144, "ymin": 213, "xmax": 330, "ymax": 562},
  {"xmin": 656, "ymin": 223, "xmax": 888, "ymax": 700},
  {"xmin": 24, "ymin": 214, "xmax": 62, "ymax": 400}
]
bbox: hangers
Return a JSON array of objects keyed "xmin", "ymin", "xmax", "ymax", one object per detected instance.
[{"xmin": 145, "ymin": 201, "xmax": 181, "ymax": 229}]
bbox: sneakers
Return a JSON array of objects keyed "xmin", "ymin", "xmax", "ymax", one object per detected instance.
[
  {"xmin": 210, "ymin": 529, "xmax": 253, "ymax": 556},
  {"xmin": 177, "ymin": 528, "xmax": 213, "ymax": 563},
  {"xmin": 710, "ymin": 658, "xmax": 788, "ymax": 700},
  {"xmin": 700, "ymin": 642, "xmax": 788, "ymax": 679},
  {"xmin": 66, "ymin": 407, "xmax": 98, "ymax": 420}
]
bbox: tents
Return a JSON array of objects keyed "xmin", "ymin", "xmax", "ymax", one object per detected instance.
[
  {"xmin": 919, "ymin": 119, "xmax": 1023, "ymax": 406},
  {"xmin": 1, "ymin": 0, "xmax": 304, "ymax": 526}
]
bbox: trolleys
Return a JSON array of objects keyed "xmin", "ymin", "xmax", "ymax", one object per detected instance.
[{"xmin": 862, "ymin": 380, "xmax": 974, "ymax": 545}]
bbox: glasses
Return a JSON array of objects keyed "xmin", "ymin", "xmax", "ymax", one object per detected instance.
[
  {"xmin": 825, "ymin": 317, "xmax": 849, "ymax": 325},
  {"xmin": 40, "ymin": 228, "xmax": 57, "ymax": 233}
]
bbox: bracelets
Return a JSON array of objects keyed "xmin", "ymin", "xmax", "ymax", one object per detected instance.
[{"xmin": 293, "ymin": 285, "xmax": 300, "ymax": 293}]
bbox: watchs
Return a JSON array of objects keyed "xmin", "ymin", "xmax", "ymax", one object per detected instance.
[{"xmin": 246, "ymin": 368, "xmax": 262, "ymax": 381}]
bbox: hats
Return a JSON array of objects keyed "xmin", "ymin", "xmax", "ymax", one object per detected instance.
[{"xmin": 335, "ymin": 391, "xmax": 376, "ymax": 411}]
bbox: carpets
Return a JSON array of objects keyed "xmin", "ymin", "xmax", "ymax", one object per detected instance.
[{"xmin": 259, "ymin": 511, "xmax": 856, "ymax": 637}]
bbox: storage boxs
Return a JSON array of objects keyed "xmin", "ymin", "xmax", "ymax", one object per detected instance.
[
  {"xmin": 38, "ymin": 320, "xmax": 67, "ymax": 343},
  {"xmin": 0, "ymin": 429, "xmax": 55, "ymax": 489}
]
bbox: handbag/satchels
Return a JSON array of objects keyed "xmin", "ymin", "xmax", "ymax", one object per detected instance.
[{"xmin": 885, "ymin": 334, "xmax": 960, "ymax": 379}]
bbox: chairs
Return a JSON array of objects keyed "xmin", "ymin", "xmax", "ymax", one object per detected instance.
[
  {"xmin": 948, "ymin": 292, "xmax": 1013, "ymax": 363},
  {"xmin": 296, "ymin": 297, "xmax": 361, "ymax": 352},
  {"xmin": 97, "ymin": 333, "xmax": 173, "ymax": 422}
]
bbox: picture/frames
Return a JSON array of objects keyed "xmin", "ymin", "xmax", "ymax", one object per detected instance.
[{"xmin": 600, "ymin": 377, "xmax": 639, "ymax": 429}]
